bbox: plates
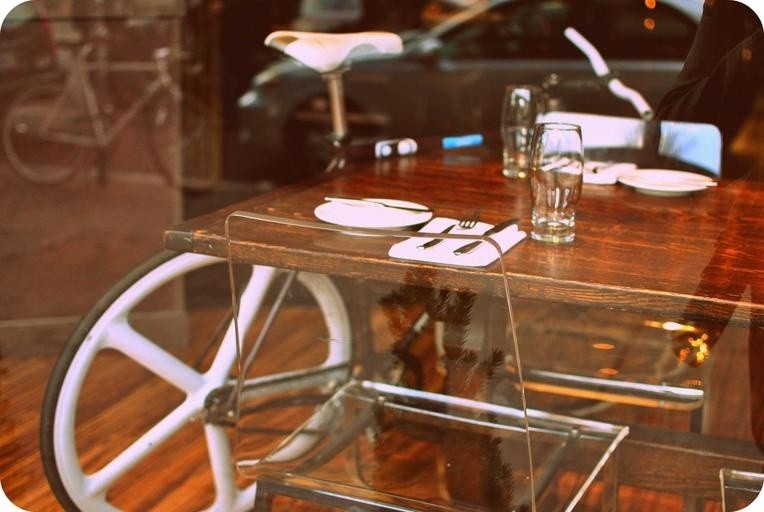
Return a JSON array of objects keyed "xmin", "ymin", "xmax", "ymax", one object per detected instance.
[
  {"xmin": 311, "ymin": 193, "xmax": 432, "ymax": 237},
  {"xmin": 618, "ymin": 168, "xmax": 714, "ymax": 199}
]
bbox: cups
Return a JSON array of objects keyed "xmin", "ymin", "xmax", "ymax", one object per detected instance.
[
  {"xmin": 500, "ymin": 80, "xmax": 545, "ymax": 182},
  {"xmin": 530, "ymin": 120, "xmax": 581, "ymax": 243}
]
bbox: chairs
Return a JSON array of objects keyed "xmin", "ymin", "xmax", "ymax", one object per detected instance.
[
  {"xmin": 718, "ymin": 468, "xmax": 764, "ymax": 512},
  {"xmin": 502, "ymin": 110, "xmax": 726, "ymax": 512},
  {"xmin": 224, "ymin": 211, "xmax": 631, "ymax": 512}
]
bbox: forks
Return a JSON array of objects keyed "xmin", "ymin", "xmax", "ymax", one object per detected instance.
[{"xmin": 413, "ymin": 212, "xmax": 482, "ymax": 248}]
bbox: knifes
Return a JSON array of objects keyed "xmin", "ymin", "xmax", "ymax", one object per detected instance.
[
  {"xmin": 452, "ymin": 217, "xmax": 520, "ymax": 257},
  {"xmin": 323, "ymin": 194, "xmax": 434, "ymax": 217}
]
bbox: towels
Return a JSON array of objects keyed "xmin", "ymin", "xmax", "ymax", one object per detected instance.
[
  {"xmin": 388, "ymin": 219, "xmax": 526, "ymax": 267},
  {"xmin": 568, "ymin": 159, "xmax": 635, "ymax": 185}
]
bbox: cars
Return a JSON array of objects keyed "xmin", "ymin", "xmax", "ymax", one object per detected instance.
[{"xmin": 240, "ymin": 1, "xmax": 705, "ymax": 181}]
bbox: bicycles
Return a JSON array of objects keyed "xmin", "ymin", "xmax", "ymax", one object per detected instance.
[
  {"xmin": 1, "ymin": 19, "xmax": 220, "ymax": 195},
  {"xmin": 41, "ymin": 32, "xmax": 712, "ymax": 512}
]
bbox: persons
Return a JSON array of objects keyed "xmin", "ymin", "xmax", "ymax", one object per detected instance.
[{"xmin": 637, "ymin": 1, "xmax": 764, "ymax": 453}]
